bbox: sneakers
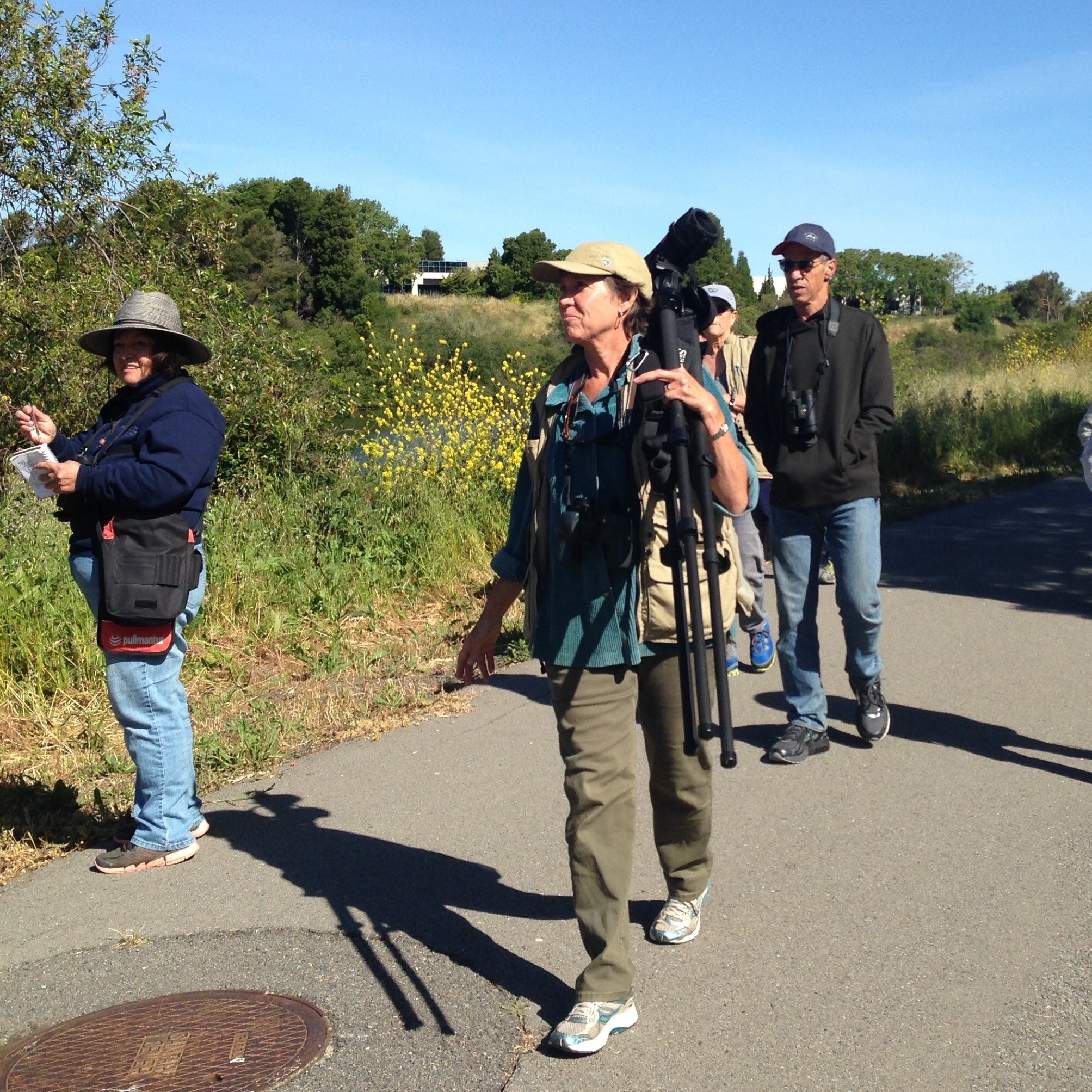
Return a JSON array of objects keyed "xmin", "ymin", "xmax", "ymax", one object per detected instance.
[
  {"xmin": 94, "ymin": 838, "xmax": 199, "ymax": 873},
  {"xmin": 848, "ymin": 674, "xmax": 891, "ymax": 742},
  {"xmin": 769, "ymin": 721, "xmax": 831, "ymax": 763},
  {"xmin": 549, "ymin": 990, "xmax": 640, "ymax": 1053},
  {"xmin": 649, "ymin": 881, "xmax": 708, "ymax": 944},
  {"xmin": 749, "ymin": 619, "xmax": 776, "ymax": 672},
  {"xmin": 112, "ymin": 816, "xmax": 209, "ymax": 843},
  {"xmin": 726, "ymin": 637, "xmax": 738, "ymax": 676}
]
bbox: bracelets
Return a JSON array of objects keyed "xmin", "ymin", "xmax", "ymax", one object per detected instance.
[{"xmin": 728, "ymin": 390, "xmax": 736, "ymax": 409}]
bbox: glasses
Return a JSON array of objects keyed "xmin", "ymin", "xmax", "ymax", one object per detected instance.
[
  {"xmin": 559, "ymin": 395, "xmax": 624, "ymax": 451},
  {"xmin": 778, "ymin": 258, "xmax": 824, "ymax": 272},
  {"xmin": 717, "ymin": 306, "xmax": 730, "ymax": 313}
]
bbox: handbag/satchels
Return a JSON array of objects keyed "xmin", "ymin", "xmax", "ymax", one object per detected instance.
[{"xmin": 96, "ymin": 516, "xmax": 205, "ymax": 653}]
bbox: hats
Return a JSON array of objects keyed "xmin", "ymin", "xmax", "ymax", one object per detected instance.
[
  {"xmin": 78, "ymin": 290, "xmax": 211, "ymax": 366},
  {"xmin": 703, "ymin": 284, "xmax": 737, "ymax": 311},
  {"xmin": 530, "ymin": 241, "xmax": 653, "ymax": 303},
  {"xmin": 772, "ymin": 222, "xmax": 835, "ymax": 260}
]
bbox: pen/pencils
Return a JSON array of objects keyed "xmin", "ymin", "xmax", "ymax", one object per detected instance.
[{"xmin": 28, "ymin": 402, "xmax": 42, "ymax": 443}]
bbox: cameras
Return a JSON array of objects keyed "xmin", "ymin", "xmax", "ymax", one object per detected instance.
[{"xmin": 644, "ymin": 208, "xmax": 720, "ymax": 291}]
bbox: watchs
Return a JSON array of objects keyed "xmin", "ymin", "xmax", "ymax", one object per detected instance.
[{"xmin": 705, "ymin": 418, "xmax": 730, "ymax": 443}]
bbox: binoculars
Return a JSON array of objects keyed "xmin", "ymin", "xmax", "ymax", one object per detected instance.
[{"xmin": 787, "ymin": 389, "xmax": 817, "ymax": 435}]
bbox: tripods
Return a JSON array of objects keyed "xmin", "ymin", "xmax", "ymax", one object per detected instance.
[{"xmin": 655, "ymin": 272, "xmax": 738, "ymax": 770}]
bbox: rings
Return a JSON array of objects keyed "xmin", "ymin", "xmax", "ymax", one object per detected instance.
[{"xmin": 667, "ymin": 383, "xmax": 671, "ymax": 391}]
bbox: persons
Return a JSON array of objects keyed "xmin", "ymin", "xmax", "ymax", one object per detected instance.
[
  {"xmin": 456, "ymin": 245, "xmax": 761, "ymax": 1053},
  {"xmin": 743, "ymin": 223, "xmax": 893, "ymax": 765},
  {"xmin": 1078, "ymin": 403, "xmax": 1092, "ymax": 494},
  {"xmin": 695, "ymin": 282, "xmax": 774, "ymax": 670},
  {"xmin": 15, "ymin": 288, "xmax": 227, "ymax": 874}
]
